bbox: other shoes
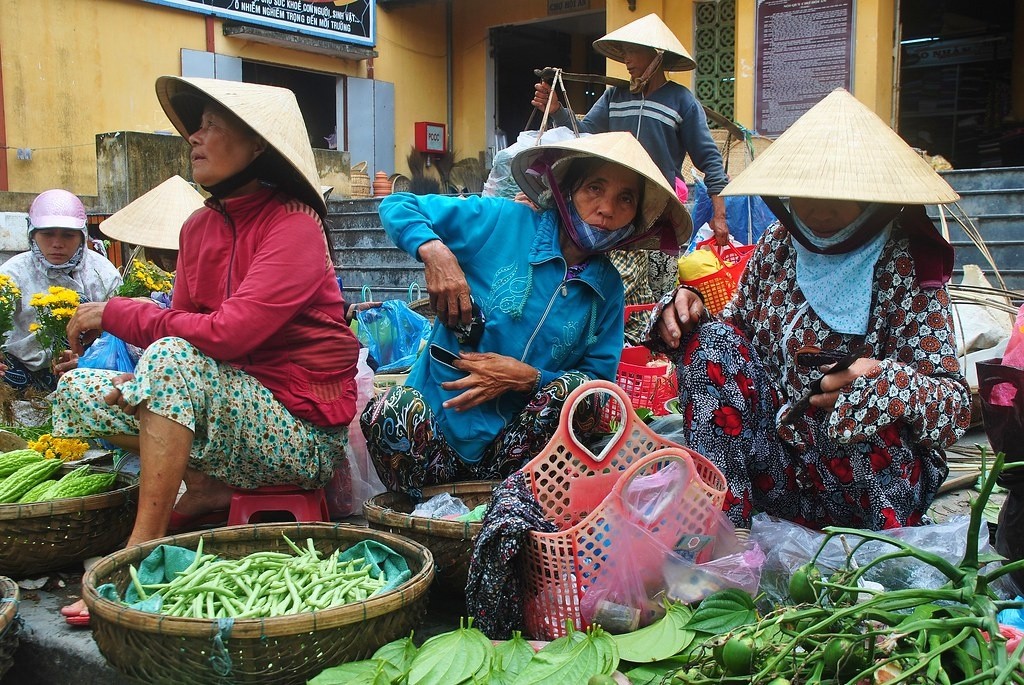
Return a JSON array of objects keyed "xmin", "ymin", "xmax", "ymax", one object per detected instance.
[
  {"xmin": 65, "ymin": 616, "xmax": 90, "ymax": 625},
  {"xmin": 168, "ymin": 510, "xmax": 228, "ymax": 530}
]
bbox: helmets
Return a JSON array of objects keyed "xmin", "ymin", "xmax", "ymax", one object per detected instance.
[{"xmin": 25, "ymin": 189, "xmax": 89, "ymax": 243}]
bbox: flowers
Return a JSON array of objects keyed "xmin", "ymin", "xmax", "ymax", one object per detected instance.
[
  {"xmin": 107, "ymin": 255, "xmax": 180, "ymax": 301},
  {"xmin": 30, "ymin": 286, "xmax": 84, "ymax": 375},
  {"xmin": 0, "ymin": 275, "xmax": 25, "ymax": 362}
]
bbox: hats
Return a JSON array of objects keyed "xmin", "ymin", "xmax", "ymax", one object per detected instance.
[
  {"xmin": 155, "ymin": 75, "xmax": 328, "ymax": 218},
  {"xmin": 592, "ymin": 12, "xmax": 698, "ymax": 72},
  {"xmin": 321, "ymin": 186, "xmax": 333, "ymax": 201},
  {"xmin": 717, "ymin": 86, "xmax": 960, "ymax": 204},
  {"xmin": 511, "ymin": 131, "xmax": 694, "ymax": 250},
  {"xmin": 99, "ymin": 174, "xmax": 206, "ymax": 250}
]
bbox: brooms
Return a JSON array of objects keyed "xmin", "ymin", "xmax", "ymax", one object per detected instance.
[{"xmin": 405, "ymin": 145, "xmax": 492, "ymax": 196}]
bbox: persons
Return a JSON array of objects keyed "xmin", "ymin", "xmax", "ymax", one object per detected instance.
[
  {"xmin": 638, "ymin": 87, "xmax": 972, "ymax": 554},
  {"xmin": 531, "ymin": 11, "xmax": 730, "ymax": 248},
  {"xmin": 359, "ymin": 131, "xmax": 693, "ymax": 508},
  {"xmin": 0, "ymin": 188, "xmax": 126, "ymax": 402},
  {"xmin": 54, "ymin": 75, "xmax": 381, "ymax": 629}
]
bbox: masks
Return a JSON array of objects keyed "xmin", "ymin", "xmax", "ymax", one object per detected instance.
[{"xmin": 561, "ymin": 189, "xmax": 643, "ymax": 254}]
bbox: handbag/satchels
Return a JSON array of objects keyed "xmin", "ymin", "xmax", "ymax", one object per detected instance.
[
  {"xmin": 580, "ymin": 460, "xmax": 766, "ymax": 635},
  {"xmin": 975, "ymin": 358, "xmax": 1024, "ymax": 561}
]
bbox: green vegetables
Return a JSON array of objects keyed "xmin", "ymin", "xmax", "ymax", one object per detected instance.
[{"xmin": 307, "ymin": 591, "xmax": 766, "ymax": 685}]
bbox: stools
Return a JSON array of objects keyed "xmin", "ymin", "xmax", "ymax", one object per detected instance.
[{"xmin": 225, "ymin": 483, "xmax": 331, "ymax": 526}]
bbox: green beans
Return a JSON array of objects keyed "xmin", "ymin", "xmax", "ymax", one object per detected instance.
[{"xmin": 131, "ymin": 535, "xmax": 389, "ymax": 620}]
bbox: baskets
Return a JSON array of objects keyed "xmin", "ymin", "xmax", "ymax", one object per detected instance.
[
  {"xmin": 678, "ymin": 235, "xmax": 757, "ymax": 316},
  {"xmin": 616, "ymin": 303, "xmax": 679, "ymax": 416},
  {"xmin": 406, "ymin": 282, "xmax": 436, "ymax": 324},
  {"xmin": 362, "ymin": 479, "xmax": 504, "ymax": 599},
  {"xmin": 518, "ymin": 380, "xmax": 728, "ymax": 641},
  {"xmin": 0, "ymin": 463, "xmax": 140, "ymax": 581},
  {"xmin": 0, "ymin": 576, "xmax": 21, "ymax": 681},
  {"xmin": 81, "ymin": 521, "xmax": 438, "ymax": 685}
]
